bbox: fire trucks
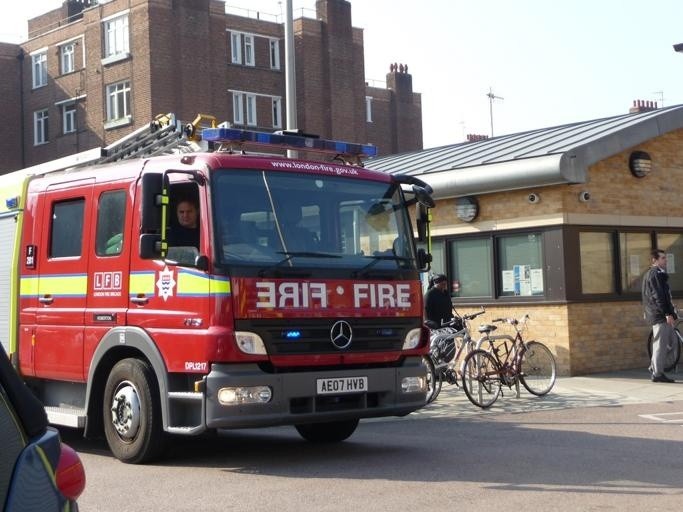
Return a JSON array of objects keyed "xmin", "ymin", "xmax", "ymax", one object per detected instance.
[{"xmin": 1, "ymin": 107, "xmax": 435, "ymax": 464}]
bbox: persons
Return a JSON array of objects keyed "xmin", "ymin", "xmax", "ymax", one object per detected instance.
[
  {"xmin": 423, "ymin": 273, "xmax": 455, "ymax": 370},
  {"xmin": 641, "ymin": 249, "xmax": 676, "ymax": 382},
  {"xmin": 162, "ymin": 197, "xmax": 200, "ymax": 255}
]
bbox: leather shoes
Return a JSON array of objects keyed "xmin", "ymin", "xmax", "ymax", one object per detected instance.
[{"xmin": 651, "ymin": 373, "xmax": 674, "ymax": 383}]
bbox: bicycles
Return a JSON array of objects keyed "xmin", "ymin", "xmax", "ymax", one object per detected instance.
[
  {"xmin": 644, "ymin": 306, "xmax": 682, "ymax": 373},
  {"xmin": 413, "ymin": 305, "xmax": 562, "ymax": 408}
]
